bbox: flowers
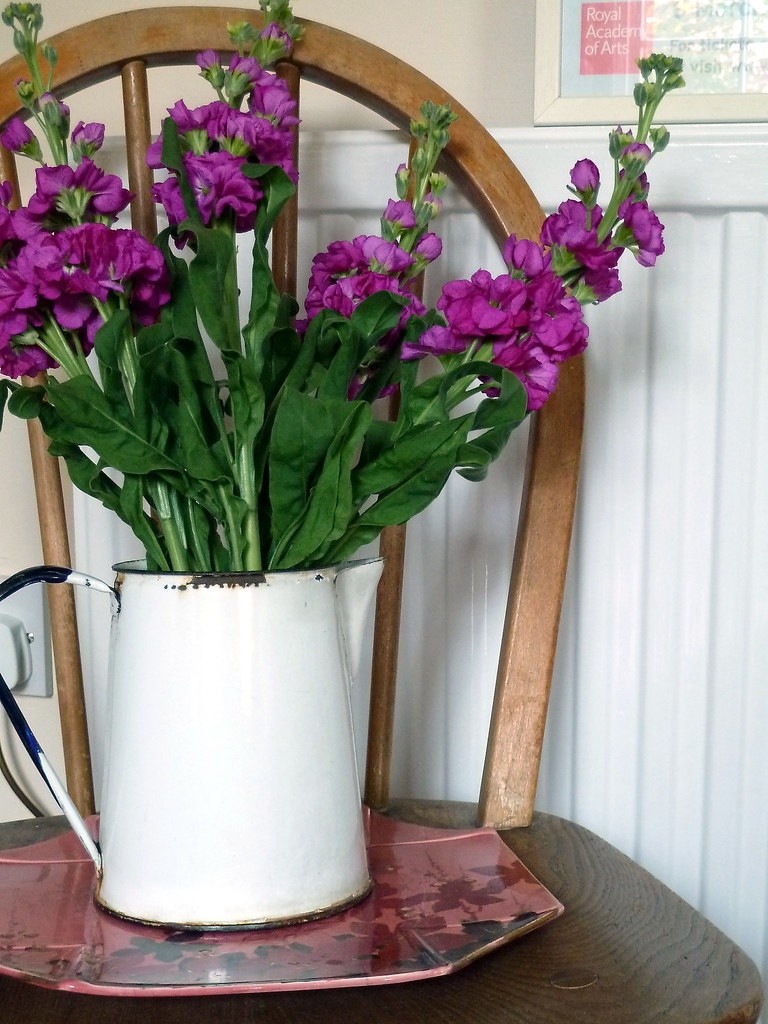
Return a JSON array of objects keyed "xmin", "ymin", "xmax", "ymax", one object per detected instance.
[{"xmin": 0, "ymin": 0, "xmax": 685, "ymax": 577}]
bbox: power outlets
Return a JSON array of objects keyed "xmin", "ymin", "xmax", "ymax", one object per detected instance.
[{"xmin": 0, "ymin": 576, "xmax": 53, "ymax": 697}]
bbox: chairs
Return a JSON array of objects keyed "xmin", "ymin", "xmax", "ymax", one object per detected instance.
[{"xmin": 0, "ymin": 7, "xmax": 764, "ymax": 1024}]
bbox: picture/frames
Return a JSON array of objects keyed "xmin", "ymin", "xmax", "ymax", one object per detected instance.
[{"xmin": 533, "ymin": 0, "xmax": 768, "ymax": 127}]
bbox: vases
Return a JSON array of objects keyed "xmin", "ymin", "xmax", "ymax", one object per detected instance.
[{"xmin": 0, "ymin": 553, "xmax": 386, "ymax": 929}]
